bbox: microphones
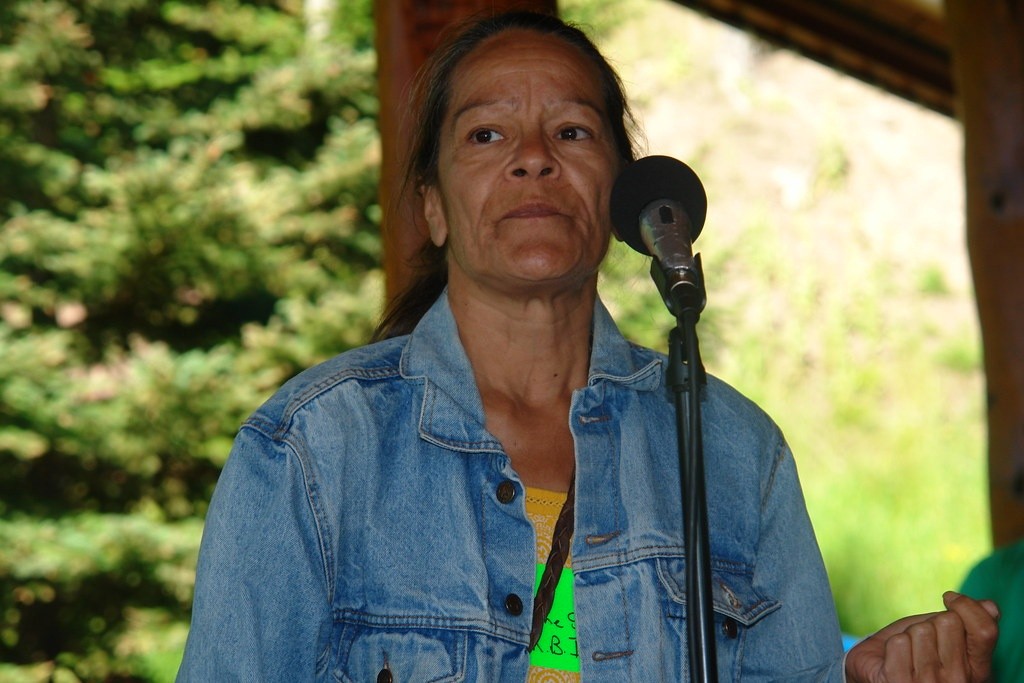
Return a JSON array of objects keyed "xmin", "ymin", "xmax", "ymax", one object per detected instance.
[{"xmin": 610, "ymin": 155, "xmax": 707, "ymax": 322}]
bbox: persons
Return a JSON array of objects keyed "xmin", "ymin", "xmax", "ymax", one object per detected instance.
[{"xmin": 172, "ymin": 12, "xmax": 1001, "ymax": 683}]
